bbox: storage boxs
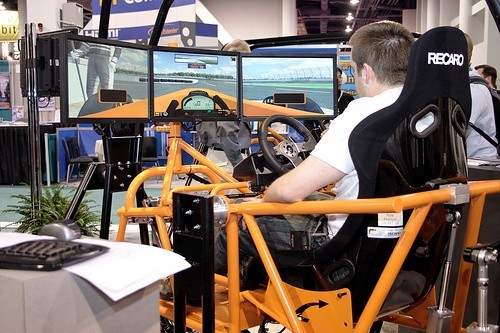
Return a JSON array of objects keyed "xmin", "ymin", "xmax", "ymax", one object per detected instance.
[{"xmin": 0, "ymin": 227, "xmax": 161, "ymax": 333}]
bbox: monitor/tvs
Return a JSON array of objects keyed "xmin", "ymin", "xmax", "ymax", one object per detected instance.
[{"xmin": 20, "ymin": 28, "xmax": 337, "ymax": 124}]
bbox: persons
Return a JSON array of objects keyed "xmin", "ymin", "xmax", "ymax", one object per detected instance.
[
  {"xmin": 465, "ymin": 32, "xmax": 498, "ymax": 167},
  {"xmin": 214, "ymin": 20, "xmax": 415, "ymax": 277},
  {"xmin": 71, "ymin": 42, "xmax": 122, "ymax": 97},
  {"xmin": 303, "ymin": 67, "xmax": 355, "ymax": 143},
  {"xmin": 474, "ymin": 64, "xmax": 500, "ymax": 157}
]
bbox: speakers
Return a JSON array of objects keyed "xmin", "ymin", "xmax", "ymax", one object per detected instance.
[{"xmin": 62, "ymin": 3, "xmax": 84, "ymax": 28}]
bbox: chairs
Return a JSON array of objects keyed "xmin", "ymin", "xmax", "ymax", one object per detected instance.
[{"xmin": 235, "ymin": 27, "xmax": 472, "ymax": 324}]
